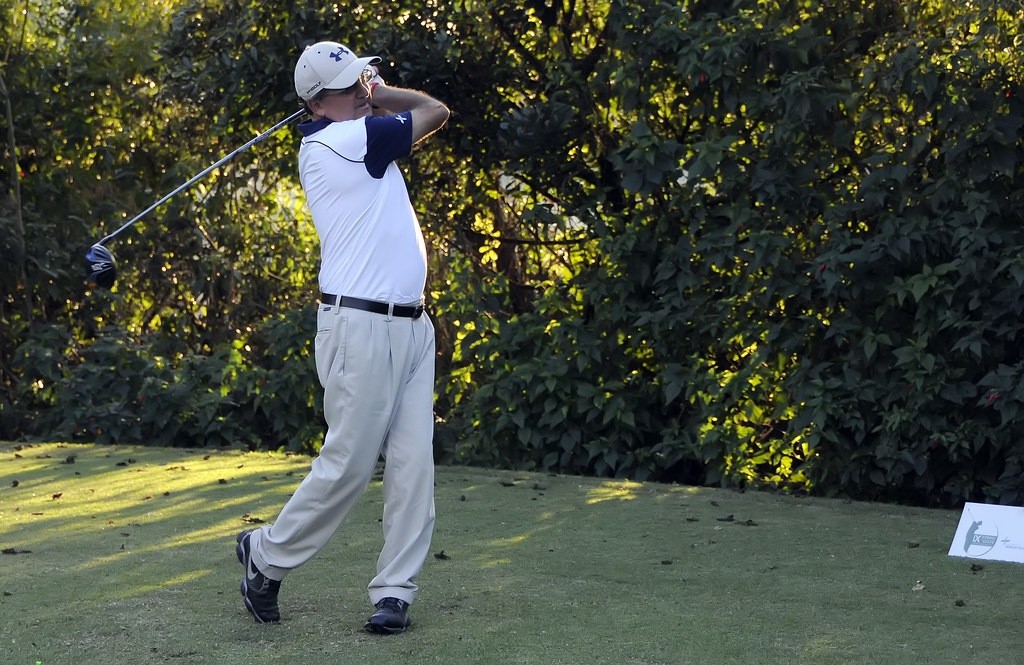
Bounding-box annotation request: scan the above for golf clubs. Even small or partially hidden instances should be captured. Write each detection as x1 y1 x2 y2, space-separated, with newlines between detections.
85 103 313 275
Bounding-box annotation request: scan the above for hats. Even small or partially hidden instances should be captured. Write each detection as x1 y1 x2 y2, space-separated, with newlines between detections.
293 41 382 108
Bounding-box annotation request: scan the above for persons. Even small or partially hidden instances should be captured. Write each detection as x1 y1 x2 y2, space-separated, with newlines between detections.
236 42 451 634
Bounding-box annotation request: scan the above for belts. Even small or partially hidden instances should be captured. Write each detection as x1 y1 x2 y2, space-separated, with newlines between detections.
319 293 426 319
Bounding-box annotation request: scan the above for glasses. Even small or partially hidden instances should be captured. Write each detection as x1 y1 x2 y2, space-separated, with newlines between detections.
319 67 373 98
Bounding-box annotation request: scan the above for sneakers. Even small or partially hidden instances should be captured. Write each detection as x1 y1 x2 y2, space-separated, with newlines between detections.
236 529 282 625
364 597 412 633
